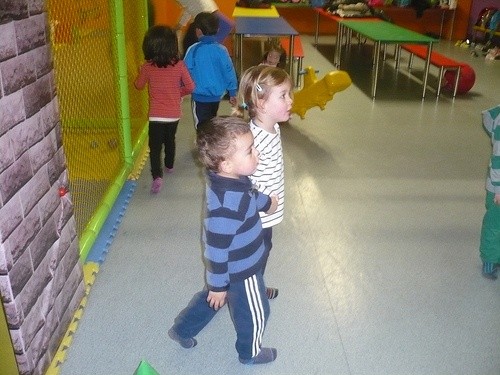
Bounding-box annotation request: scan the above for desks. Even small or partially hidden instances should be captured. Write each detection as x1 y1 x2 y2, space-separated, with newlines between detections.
232 5 280 59
313 7 385 66
336 20 440 97
235 16 300 83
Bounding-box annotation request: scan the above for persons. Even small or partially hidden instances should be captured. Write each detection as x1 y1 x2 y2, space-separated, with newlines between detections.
168 115 278 365
176 0 220 61
132 24 195 195
182 11 241 130
481 105 500 273
230 64 292 300
256 37 287 68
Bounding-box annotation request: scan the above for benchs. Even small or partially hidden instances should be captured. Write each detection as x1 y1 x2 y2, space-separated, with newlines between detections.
472 26 500 48
396 43 464 97
279 35 304 87
